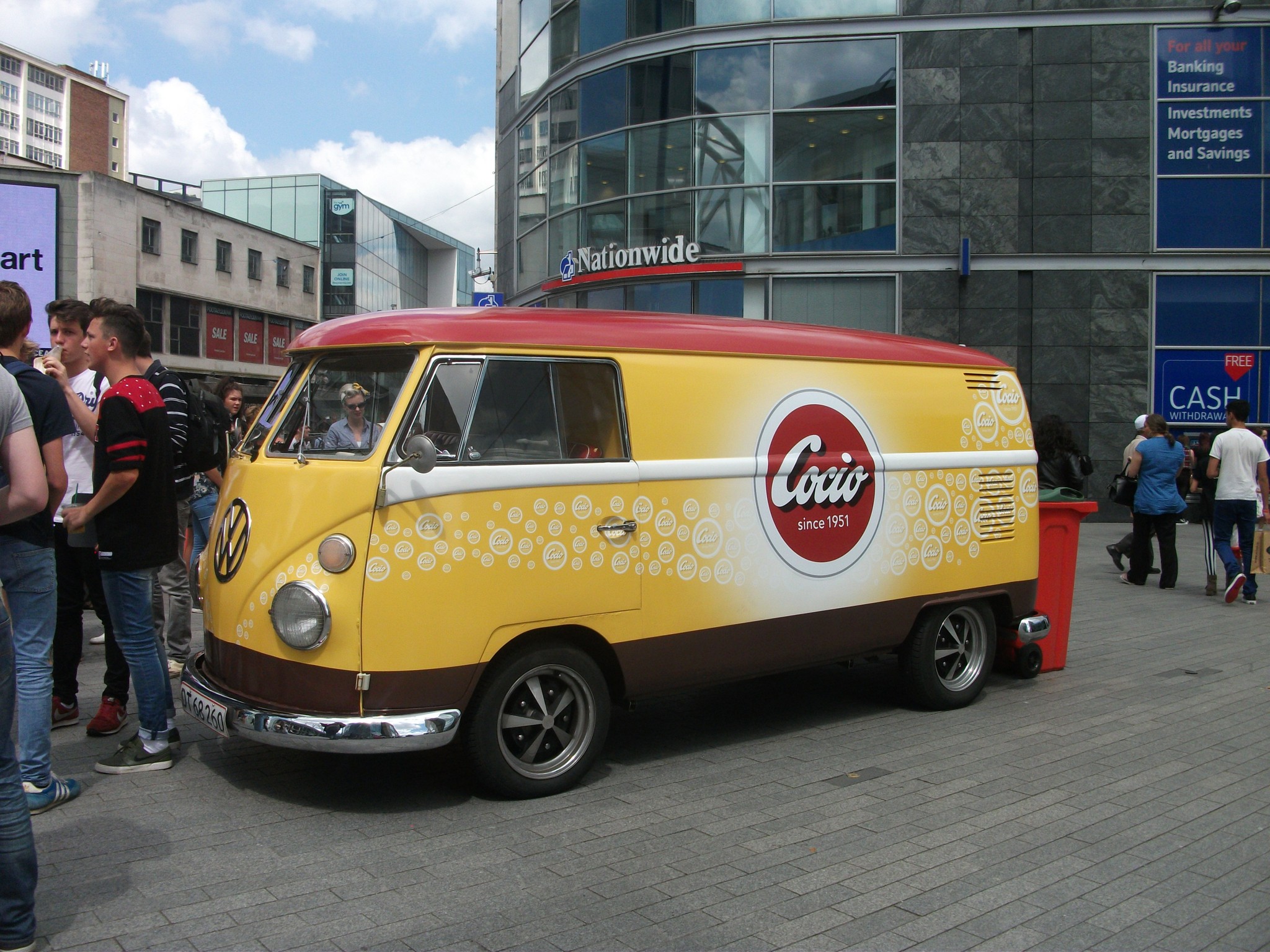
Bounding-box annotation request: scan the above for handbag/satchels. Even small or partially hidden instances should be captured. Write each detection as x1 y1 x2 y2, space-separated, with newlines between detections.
1077 455 1093 475
1250 522 1270 575
1107 461 1139 506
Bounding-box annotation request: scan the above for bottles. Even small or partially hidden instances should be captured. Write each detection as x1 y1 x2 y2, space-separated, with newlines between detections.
48 344 63 362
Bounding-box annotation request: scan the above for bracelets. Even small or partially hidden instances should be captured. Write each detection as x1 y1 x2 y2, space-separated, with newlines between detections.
1262 510 1269 513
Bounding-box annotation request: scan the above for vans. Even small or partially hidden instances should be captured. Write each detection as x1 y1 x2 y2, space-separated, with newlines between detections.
176 301 1052 800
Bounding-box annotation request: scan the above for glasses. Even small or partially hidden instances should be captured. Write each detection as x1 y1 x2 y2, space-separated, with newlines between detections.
345 402 365 410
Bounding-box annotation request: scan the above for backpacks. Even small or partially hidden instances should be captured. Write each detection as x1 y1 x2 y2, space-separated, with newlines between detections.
156 371 232 473
317 438 325 449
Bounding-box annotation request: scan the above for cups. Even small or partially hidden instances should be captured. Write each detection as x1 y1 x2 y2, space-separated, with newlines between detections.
62 502 97 547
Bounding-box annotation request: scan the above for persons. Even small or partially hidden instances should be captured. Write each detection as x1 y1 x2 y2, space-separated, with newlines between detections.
1034 414 1083 490
286 421 310 451
0 280 282 952
1106 400 1270 604
325 383 380 449
314 434 324 449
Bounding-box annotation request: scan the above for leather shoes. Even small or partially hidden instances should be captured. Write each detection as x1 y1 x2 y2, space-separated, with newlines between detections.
1107 544 1124 571
1148 566 1160 574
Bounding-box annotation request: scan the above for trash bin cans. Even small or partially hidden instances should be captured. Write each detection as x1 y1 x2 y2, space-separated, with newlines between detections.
993 500 1097 680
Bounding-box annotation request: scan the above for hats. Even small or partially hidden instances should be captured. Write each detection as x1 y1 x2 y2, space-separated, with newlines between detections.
1134 414 1149 430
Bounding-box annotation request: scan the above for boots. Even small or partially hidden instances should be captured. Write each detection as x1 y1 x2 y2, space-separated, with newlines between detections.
1205 575 1217 595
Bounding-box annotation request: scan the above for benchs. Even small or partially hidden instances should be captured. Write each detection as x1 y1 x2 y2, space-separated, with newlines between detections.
421 431 602 459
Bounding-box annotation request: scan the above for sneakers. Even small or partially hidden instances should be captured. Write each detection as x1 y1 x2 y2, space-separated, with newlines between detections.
94 738 172 772
119 726 182 751
23 770 81 815
85 694 129 736
51 696 79 728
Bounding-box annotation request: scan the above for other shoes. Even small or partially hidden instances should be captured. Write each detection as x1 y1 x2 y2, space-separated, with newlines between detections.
168 659 185 678
192 602 203 613
1175 518 1189 525
1242 595 1256 604
1224 573 1246 603
89 633 105 643
1120 573 1146 586
